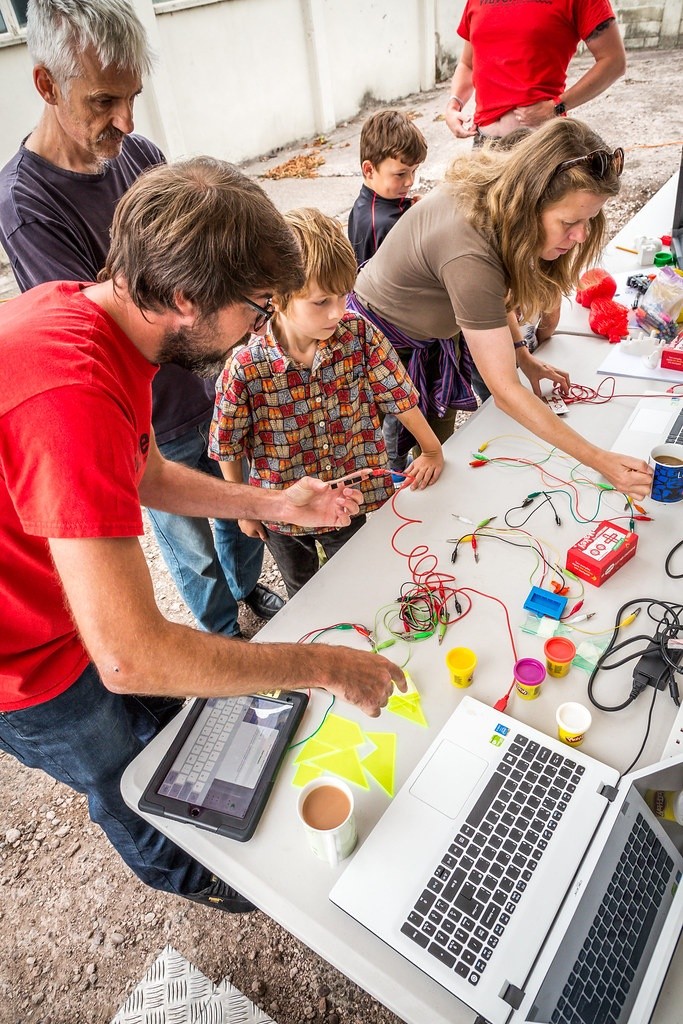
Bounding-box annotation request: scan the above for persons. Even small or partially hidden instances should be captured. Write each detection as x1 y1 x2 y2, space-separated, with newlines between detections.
208 208 444 599
0 157 406 913
443 0 627 153
0 0 286 645
347 119 656 499
348 111 428 273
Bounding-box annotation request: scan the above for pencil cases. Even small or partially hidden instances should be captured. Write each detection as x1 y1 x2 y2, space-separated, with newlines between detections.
634 265 683 336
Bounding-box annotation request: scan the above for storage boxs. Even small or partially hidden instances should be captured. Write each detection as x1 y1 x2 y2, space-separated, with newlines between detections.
661 330 683 371
567 520 638 588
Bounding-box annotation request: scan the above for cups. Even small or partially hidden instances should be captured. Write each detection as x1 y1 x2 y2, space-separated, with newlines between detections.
514 659 546 700
446 646 477 689
649 444 683 504
297 776 358 865
557 704 591 746
545 637 575 677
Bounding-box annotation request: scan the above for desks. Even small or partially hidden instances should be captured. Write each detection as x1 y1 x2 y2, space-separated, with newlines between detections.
115 171 683 1024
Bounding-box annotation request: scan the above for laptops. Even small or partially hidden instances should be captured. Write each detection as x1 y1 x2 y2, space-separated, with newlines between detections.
610 390 683 462
327 694 683 1024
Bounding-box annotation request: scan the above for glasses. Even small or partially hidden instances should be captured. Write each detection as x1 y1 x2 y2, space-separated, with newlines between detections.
236 291 275 332
553 147 625 181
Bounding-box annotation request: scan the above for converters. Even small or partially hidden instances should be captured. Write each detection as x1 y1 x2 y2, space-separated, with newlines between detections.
632 631 683 692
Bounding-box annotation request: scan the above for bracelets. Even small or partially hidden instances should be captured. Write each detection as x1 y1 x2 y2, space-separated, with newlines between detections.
514 339 528 350
449 96 464 112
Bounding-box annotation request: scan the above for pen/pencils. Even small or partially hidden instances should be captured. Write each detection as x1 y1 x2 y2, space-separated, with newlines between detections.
616 247 638 255
636 302 678 343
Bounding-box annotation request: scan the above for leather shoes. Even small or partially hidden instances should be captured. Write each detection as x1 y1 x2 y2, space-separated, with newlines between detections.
240 582 286 620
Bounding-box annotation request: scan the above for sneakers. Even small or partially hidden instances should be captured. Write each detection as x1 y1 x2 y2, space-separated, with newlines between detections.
179 875 256 914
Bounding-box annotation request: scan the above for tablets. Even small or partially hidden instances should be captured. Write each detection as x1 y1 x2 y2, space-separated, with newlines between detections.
138 690 309 843
